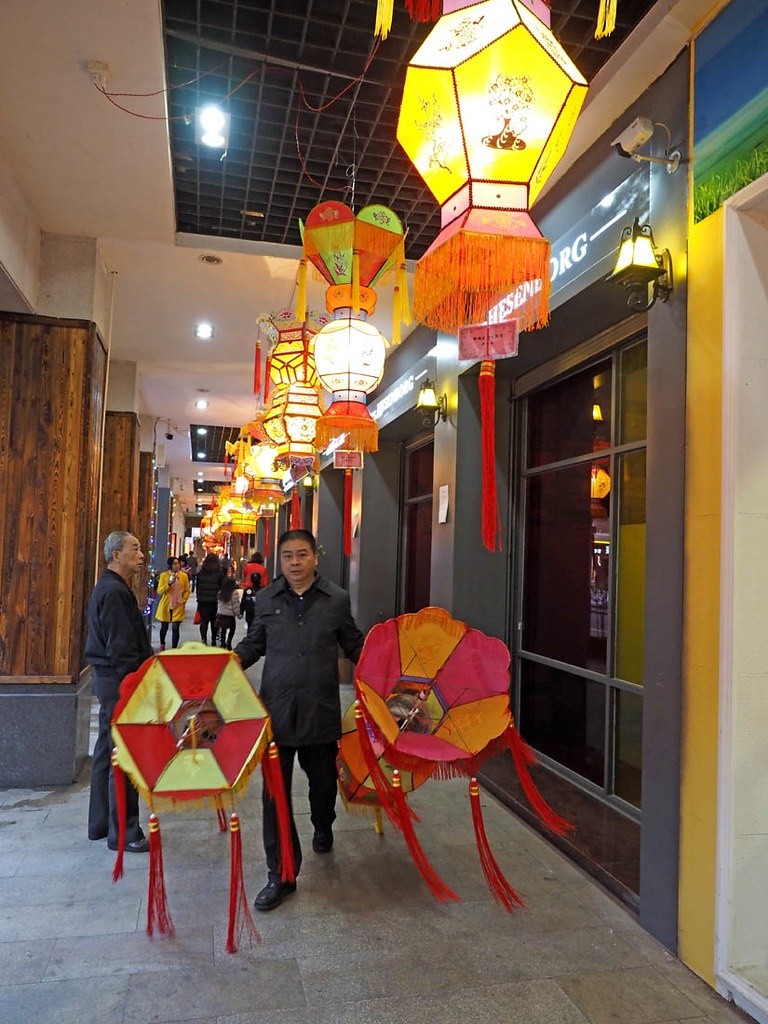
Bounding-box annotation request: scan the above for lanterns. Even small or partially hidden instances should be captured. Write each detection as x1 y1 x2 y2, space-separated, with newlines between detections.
200 200 405 557
111 642 298 954
590 439 611 522
336 607 576 913
374 0 620 551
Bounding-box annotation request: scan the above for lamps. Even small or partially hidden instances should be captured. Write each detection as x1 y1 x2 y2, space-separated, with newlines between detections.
413 378 447 428
605 217 673 313
302 471 317 494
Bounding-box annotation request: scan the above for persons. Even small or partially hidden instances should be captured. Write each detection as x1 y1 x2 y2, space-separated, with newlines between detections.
178 551 248 593
243 552 268 590
195 553 243 651
240 572 266 628
157 557 190 651
83 531 156 853
231 528 366 910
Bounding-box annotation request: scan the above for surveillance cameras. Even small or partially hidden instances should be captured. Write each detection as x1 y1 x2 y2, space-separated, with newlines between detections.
165 432 174 440
609 115 655 159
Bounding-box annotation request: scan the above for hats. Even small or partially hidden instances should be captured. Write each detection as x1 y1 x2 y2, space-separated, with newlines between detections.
251 574 260 581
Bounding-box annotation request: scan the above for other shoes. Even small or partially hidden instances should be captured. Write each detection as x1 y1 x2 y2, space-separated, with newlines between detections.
159 642 165 651
226 642 232 651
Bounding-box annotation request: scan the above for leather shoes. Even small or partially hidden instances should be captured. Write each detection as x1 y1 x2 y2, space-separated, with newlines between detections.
255 877 296 910
108 837 150 852
312 824 333 853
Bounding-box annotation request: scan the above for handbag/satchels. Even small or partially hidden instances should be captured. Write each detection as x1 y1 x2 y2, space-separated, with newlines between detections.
194 610 200 624
